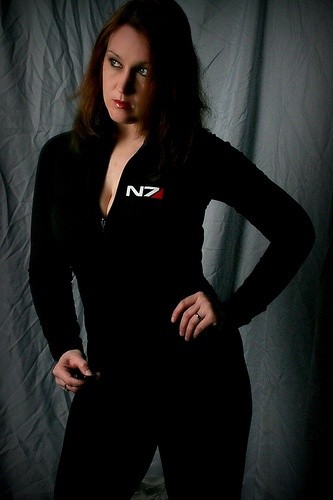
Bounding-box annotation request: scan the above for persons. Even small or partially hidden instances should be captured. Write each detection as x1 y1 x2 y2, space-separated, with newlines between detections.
27 0 319 500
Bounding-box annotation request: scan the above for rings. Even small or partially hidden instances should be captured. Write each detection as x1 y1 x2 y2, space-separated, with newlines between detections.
64 384 70 393
195 312 202 321
